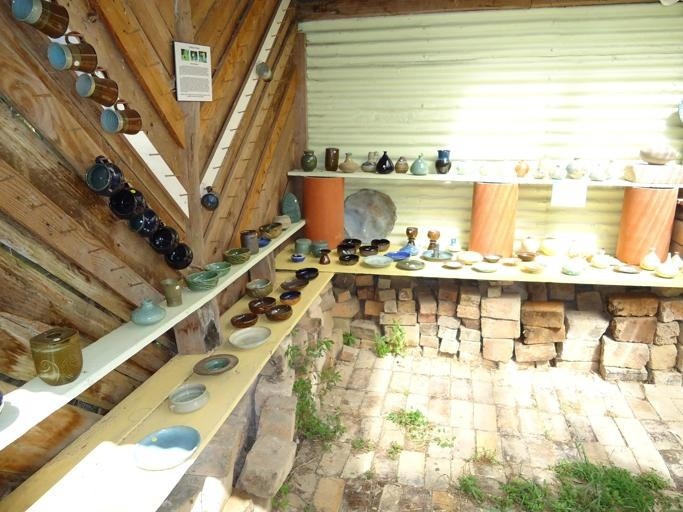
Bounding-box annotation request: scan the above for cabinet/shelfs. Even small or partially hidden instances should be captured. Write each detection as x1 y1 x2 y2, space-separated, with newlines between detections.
273 161 683 306
1 217 335 510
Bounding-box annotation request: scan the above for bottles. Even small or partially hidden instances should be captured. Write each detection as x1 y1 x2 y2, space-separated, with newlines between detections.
410 154 430 175
376 151 394 174
339 153 360 173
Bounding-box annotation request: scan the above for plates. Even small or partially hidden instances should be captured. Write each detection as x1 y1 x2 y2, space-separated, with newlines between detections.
193 353 240 376
473 262 499 274
364 254 393 268
399 260 425 271
521 263 544 274
502 257 522 266
424 250 453 262
445 261 463 269
133 426 202 472
458 251 483 264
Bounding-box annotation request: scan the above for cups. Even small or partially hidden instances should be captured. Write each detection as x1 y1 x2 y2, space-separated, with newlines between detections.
75 67 119 108
100 99 142 136
325 148 339 172
159 277 184 308
46 31 100 74
301 149 318 173
109 183 147 221
150 225 178 256
130 206 162 240
10 0 72 39
86 154 125 194
164 242 195 270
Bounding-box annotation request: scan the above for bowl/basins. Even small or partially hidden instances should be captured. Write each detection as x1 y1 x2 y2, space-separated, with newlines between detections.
278 290 303 306
230 314 259 328
359 246 378 256
205 262 233 276
247 297 277 315
484 254 502 263
281 279 309 291
257 238 271 248
229 326 273 349
184 271 219 292
291 254 305 262
337 245 357 255
266 305 293 321
295 267 319 281
371 239 391 253
259 223 283 239
517 250 537 262
246 279 274 298
166 382 211 414
386 251 411 261
342 239 362 248
222 247 251 264
338 255 359 265
